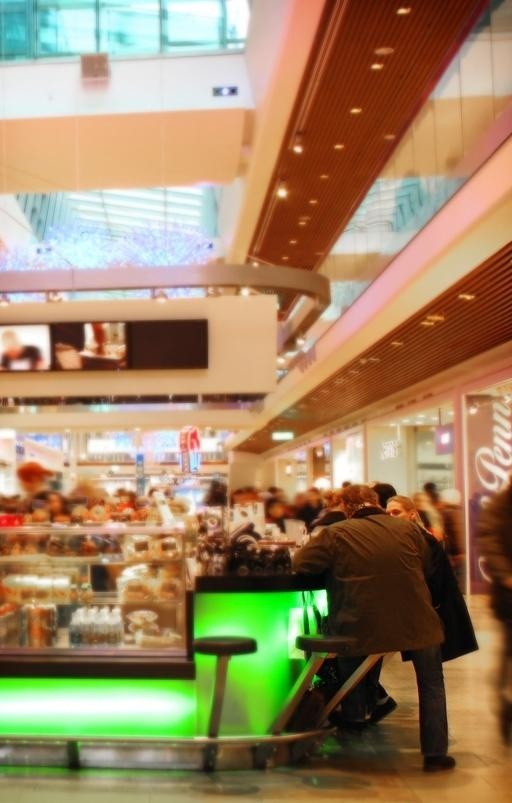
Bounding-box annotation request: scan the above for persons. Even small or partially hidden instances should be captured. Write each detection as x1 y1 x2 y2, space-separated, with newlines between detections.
1 462 190 591
476 471 512 748
204 480 479 776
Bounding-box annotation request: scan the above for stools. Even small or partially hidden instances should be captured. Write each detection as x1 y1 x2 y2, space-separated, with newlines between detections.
190 635 360 774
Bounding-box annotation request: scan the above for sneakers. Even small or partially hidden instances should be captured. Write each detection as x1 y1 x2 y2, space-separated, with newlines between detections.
367 697 396 722
425 753 455 771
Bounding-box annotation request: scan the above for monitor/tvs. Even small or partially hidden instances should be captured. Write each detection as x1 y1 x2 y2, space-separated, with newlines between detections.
0 319 208 373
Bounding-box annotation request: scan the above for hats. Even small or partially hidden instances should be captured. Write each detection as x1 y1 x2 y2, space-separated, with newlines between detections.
18 462 43 480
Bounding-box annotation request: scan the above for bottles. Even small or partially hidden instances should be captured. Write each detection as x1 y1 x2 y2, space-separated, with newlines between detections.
69 607 125 648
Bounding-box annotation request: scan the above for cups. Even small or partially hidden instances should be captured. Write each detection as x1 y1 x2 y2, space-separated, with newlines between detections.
285 520 307 543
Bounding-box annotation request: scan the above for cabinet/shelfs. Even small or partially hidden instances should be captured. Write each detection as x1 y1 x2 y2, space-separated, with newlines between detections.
0 524 191 659
